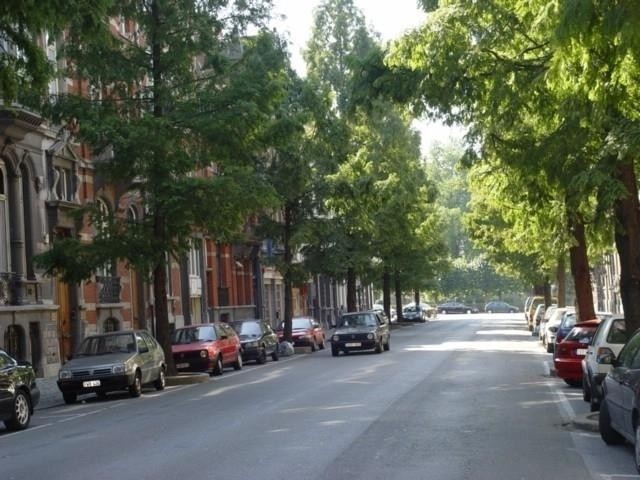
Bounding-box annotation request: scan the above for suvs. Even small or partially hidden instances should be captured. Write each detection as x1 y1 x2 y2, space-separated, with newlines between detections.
330 312 390 356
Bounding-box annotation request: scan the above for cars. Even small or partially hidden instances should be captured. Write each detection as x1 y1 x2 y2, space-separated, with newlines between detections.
485 302 519 313
373 302 434 322
228 319 279 364
523 297 640 471
436 302 479 314
0 347 39 431
274 317 325 352
171 322 244 374
56 328 165 402
